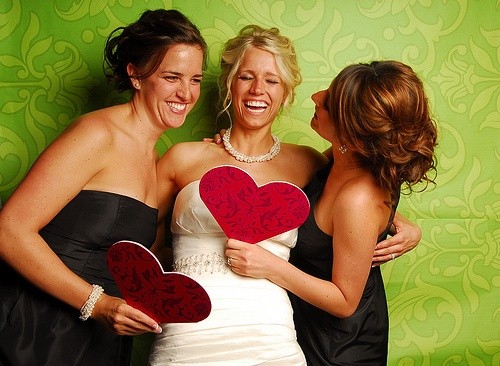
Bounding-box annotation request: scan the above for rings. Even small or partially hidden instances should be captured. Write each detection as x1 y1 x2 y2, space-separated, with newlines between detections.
391 254 395 260
227 258 231 266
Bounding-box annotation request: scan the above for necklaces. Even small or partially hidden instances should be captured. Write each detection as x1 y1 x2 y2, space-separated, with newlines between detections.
222 127 280 163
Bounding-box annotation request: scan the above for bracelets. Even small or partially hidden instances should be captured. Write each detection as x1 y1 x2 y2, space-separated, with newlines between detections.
77 283 105 322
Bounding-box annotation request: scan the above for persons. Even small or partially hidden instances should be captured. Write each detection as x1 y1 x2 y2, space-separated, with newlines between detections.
152 24 422 366
0 9 207 366
224 60 438 366
